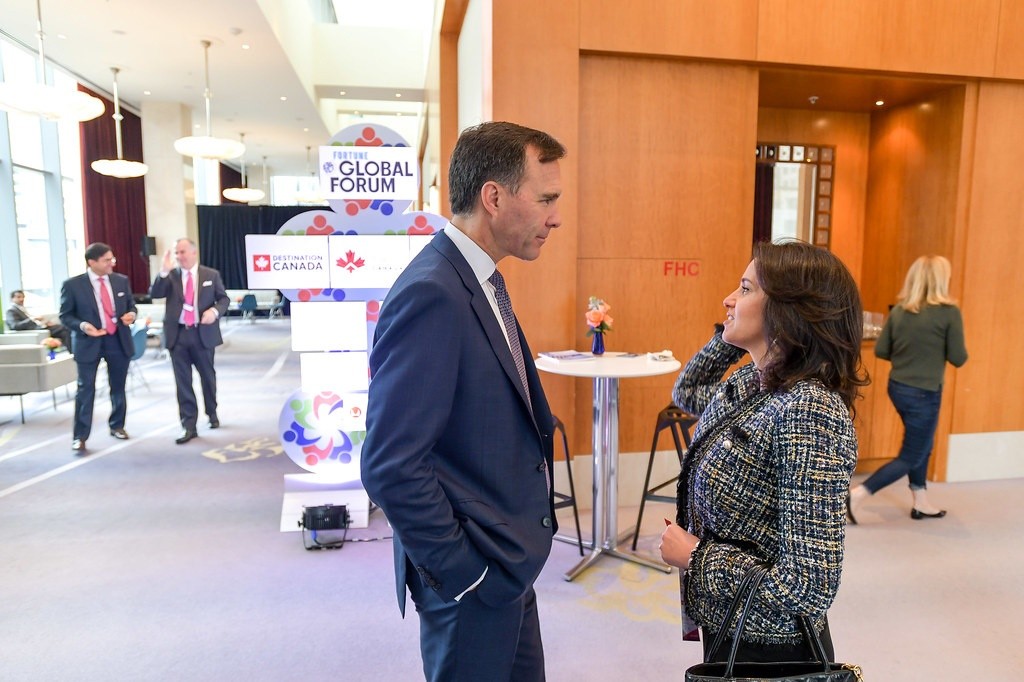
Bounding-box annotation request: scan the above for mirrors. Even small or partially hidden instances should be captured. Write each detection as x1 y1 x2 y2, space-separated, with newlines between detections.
752 141 836 256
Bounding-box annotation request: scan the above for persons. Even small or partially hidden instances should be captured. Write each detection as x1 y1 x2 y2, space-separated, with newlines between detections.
150 238 231 444
6 291 72 354
360 121 567 682
59 243 138 451
847 255 968 526
657 241 872 663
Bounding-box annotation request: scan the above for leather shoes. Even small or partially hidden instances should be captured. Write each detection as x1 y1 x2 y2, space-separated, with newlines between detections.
176 432 197 444
210 421 219 428
72 439 86 450
110 429 129 439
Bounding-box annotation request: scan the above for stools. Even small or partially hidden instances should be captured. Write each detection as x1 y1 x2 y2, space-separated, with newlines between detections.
550 414 584 558
631 401 700 550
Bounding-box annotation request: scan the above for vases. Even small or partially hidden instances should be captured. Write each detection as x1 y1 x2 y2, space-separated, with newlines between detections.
591 323 605 356
51 349 55 360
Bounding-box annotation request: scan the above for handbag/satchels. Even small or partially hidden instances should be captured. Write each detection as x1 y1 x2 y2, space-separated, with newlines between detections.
683 565 862 682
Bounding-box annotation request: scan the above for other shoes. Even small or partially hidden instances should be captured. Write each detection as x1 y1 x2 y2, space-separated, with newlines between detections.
911 508 947 520
847 489 857 524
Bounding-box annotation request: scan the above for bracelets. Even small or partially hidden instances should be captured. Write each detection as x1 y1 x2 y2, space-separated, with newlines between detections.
688 541 700 565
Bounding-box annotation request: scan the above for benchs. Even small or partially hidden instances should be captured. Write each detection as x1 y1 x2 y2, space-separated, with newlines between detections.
134 304 166 359
224 290 277 319
0 313 77 425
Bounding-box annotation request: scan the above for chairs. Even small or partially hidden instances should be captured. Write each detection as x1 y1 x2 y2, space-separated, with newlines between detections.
99 328 148 400
269 295 285 319
238 295 257 319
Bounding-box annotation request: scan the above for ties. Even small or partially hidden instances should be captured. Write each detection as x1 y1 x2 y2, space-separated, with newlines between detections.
183 271 194 327
488 268 551 501
98 278 116 335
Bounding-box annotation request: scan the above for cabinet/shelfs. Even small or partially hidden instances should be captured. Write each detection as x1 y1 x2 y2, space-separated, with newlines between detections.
849 339 905 474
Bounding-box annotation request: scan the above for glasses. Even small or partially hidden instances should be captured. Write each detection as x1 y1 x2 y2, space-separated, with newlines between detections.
94 256 116 265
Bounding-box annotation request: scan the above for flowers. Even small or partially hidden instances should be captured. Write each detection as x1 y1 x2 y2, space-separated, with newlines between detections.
40 337 63 349
585 295 614 337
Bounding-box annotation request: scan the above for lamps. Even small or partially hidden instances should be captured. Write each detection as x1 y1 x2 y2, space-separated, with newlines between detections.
0 0 105 123
90 67 148 178
174 40 247 160
222 133 265 203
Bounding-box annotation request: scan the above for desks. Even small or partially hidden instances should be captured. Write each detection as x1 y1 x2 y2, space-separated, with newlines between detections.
538 353 681 581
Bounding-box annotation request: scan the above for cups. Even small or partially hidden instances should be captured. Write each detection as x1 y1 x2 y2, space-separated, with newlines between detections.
863 311 884 340
